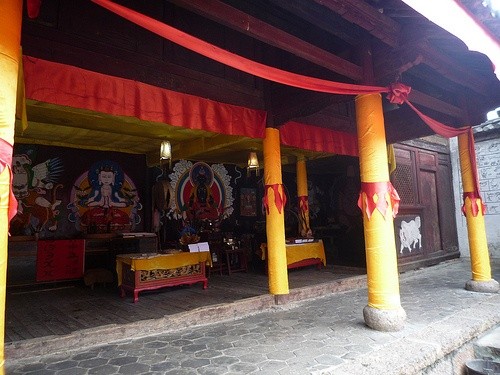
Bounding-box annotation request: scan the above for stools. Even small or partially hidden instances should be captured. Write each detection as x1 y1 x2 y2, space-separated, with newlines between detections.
223 250 249 276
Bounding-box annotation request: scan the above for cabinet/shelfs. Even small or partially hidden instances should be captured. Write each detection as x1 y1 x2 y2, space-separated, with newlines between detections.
7 233 159 297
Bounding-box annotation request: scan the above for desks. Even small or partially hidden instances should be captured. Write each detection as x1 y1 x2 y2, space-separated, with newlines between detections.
116 248 209 302
259 238 326 272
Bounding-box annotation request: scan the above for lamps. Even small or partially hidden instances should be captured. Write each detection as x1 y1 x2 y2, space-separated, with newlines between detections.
159 136 172 170
246 151 260 178
